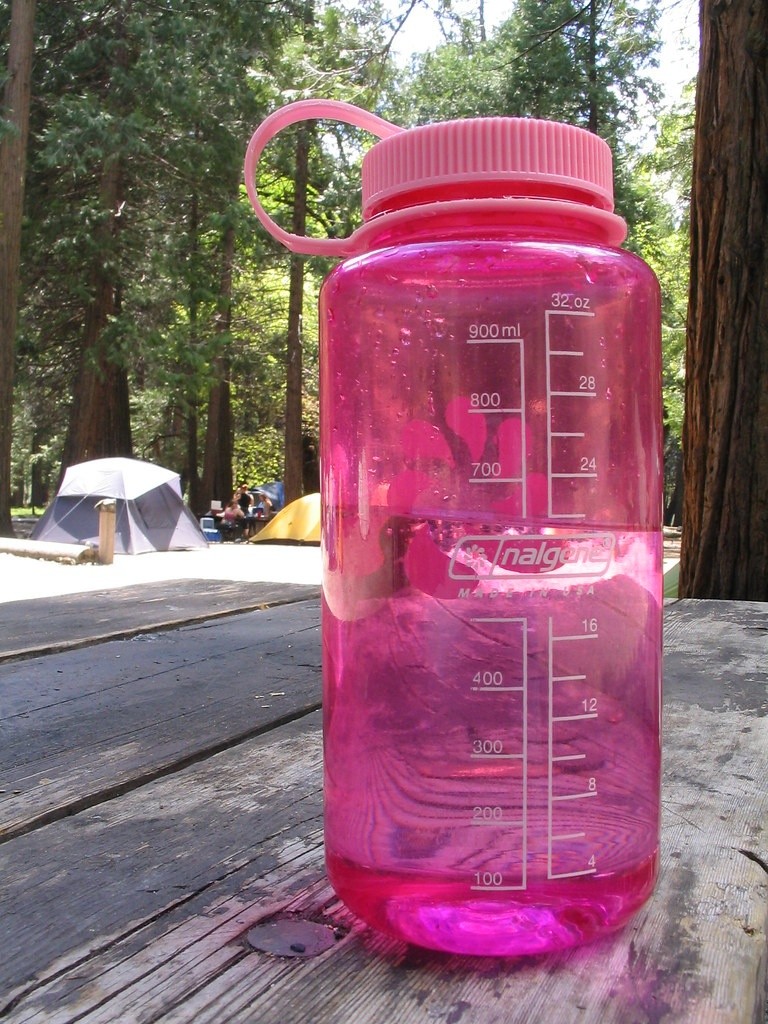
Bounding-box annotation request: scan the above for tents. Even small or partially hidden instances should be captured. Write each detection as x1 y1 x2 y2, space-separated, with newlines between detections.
249 493 321 545
29 456 211 555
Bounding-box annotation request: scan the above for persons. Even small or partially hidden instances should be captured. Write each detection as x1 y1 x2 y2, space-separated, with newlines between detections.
221 501 245 542
258 493 275 526
233 484 254 538
246 494 257 535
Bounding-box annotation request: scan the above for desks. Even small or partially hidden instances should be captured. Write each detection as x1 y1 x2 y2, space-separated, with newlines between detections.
1 580 768 1024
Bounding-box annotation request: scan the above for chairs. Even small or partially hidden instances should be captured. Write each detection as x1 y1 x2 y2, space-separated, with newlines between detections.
205 514 274 543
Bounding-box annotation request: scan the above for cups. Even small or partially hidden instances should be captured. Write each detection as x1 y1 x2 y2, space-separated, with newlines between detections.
242 99 659 954
